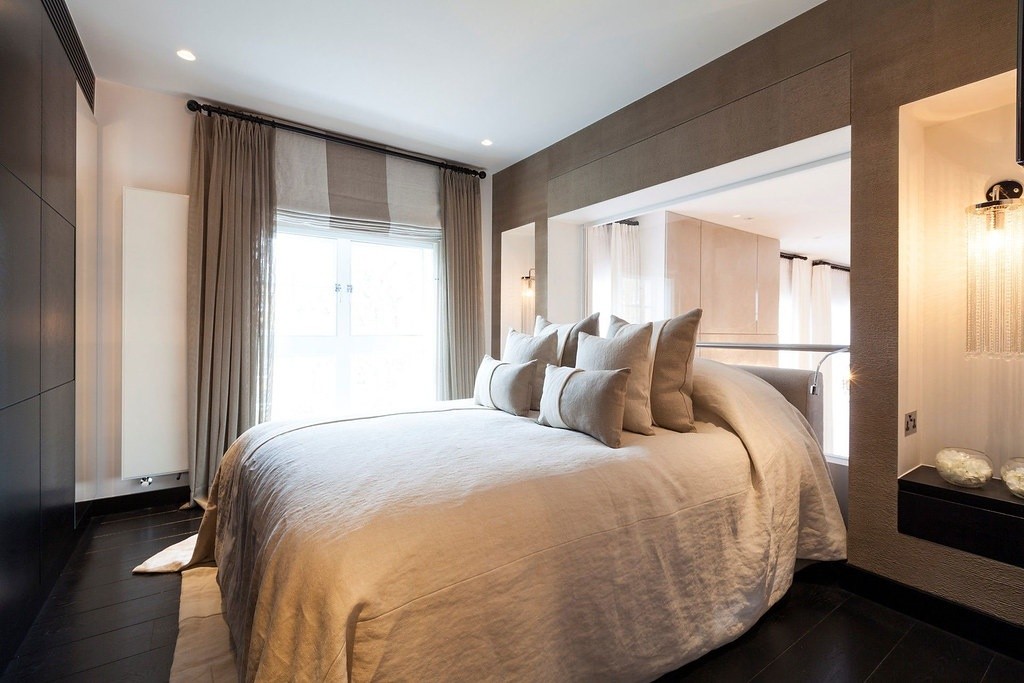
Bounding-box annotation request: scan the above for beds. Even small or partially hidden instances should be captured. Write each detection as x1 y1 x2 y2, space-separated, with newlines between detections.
127 362 849 683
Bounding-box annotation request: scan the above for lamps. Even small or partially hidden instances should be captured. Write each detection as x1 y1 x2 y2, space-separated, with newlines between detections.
521 268 535 298
964 180 1023 362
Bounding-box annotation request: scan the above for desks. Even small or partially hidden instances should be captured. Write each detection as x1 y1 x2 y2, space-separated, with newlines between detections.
897 465 1024 568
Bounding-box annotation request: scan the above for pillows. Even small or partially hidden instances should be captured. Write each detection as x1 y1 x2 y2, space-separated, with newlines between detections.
504 327 559 411
536 363 632 449
608 307 699 434
534 311 600 366
575 321 656 436
473 353 539 417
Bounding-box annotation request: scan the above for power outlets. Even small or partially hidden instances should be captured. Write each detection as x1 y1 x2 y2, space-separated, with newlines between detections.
905 410 918 438
